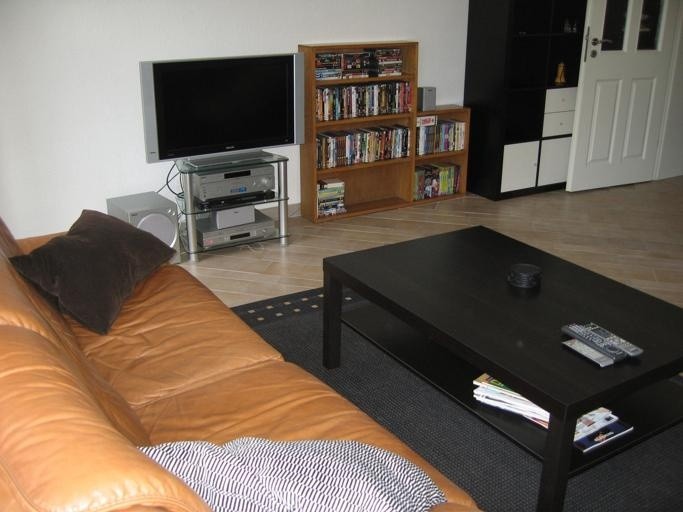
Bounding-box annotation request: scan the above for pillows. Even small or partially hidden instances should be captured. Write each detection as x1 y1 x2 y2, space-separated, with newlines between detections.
138 438 449 512
9 208 179 335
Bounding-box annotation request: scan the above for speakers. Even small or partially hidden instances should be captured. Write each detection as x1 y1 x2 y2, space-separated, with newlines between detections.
418 87 436 112
106 190 181 265
209 205 256 230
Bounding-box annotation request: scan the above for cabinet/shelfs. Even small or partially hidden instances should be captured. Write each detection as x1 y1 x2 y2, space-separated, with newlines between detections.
500 3 591 196
414 114 469 204
173 149 292 265
315 43 415 219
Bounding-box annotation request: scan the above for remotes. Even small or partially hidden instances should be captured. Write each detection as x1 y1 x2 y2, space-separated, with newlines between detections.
561 339 614 368
560 322 628 362
583 322 644 357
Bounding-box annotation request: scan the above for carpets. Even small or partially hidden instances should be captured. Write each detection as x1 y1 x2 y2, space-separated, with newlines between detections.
229 284 681 512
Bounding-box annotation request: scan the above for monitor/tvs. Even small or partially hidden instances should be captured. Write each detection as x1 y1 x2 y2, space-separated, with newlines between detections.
139 52 305 167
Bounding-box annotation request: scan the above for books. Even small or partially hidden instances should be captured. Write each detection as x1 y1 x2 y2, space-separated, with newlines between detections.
316 47 412 218
415 116 465 199
473 371 634 453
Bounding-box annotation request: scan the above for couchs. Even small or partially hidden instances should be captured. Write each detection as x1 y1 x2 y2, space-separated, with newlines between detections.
1 217 484 512
